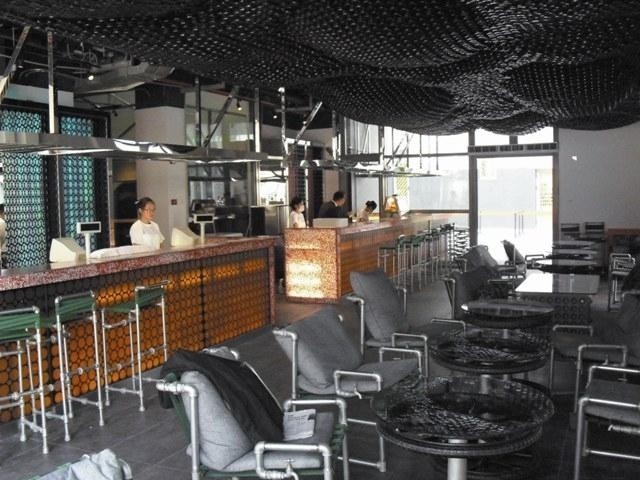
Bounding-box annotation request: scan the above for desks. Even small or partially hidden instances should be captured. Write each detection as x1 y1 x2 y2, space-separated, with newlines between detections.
546 287 630 411
373 367 558 480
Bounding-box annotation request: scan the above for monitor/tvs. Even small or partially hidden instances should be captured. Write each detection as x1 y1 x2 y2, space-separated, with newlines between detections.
49 237 84 262
171 226 201 247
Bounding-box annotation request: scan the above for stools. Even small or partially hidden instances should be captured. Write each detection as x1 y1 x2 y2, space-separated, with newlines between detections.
18 288 110 442
0 303 52 456
97 278 172 416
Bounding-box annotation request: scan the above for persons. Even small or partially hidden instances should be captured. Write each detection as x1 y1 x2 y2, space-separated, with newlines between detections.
129 198 165 252
0 204 8 250
278 196 305 295
319 191 345 218
357 201 377 222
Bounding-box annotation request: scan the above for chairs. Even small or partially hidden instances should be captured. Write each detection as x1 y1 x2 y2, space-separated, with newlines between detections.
152 345 353 479
433 322 553 377
341 267 470 382
273 303 425 475
375 228 638 337
572 362 636 479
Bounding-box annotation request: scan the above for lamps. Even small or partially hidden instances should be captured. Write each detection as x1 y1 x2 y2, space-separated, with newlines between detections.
236 97 243 111
270 109 277 119
